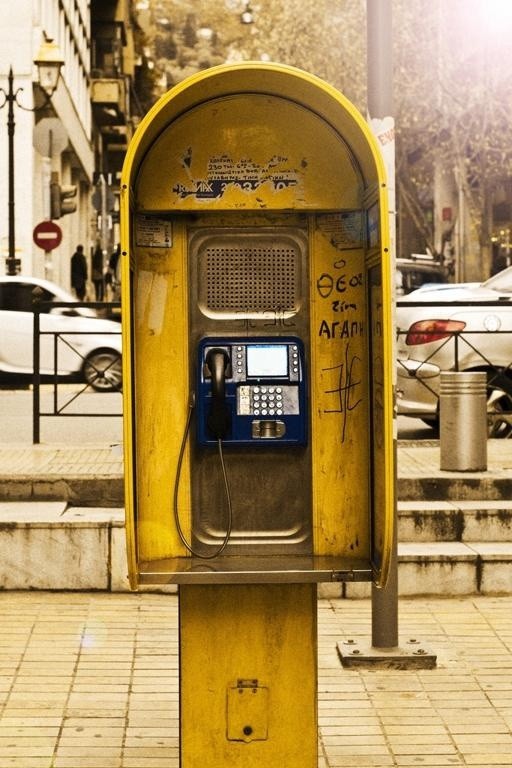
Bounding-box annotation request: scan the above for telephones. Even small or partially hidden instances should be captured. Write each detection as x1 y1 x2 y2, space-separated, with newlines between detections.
205 348 232 438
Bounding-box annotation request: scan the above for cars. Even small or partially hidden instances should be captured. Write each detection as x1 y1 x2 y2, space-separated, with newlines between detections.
396 264 512 439
0 276 126 392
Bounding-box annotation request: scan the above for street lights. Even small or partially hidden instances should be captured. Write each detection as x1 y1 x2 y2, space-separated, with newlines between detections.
1 36 65 277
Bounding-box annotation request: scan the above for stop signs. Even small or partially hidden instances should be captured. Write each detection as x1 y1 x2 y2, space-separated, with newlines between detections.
33 220 62 254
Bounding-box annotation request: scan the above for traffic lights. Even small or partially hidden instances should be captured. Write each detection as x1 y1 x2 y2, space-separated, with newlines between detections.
51 185 77 219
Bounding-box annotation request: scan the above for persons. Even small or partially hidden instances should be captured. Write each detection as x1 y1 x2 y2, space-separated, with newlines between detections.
109 241 121 277
69 244 89 305
88 238 108 303
